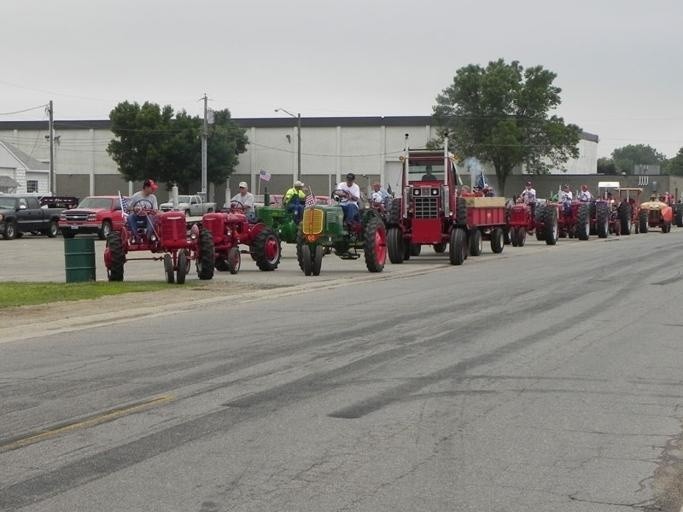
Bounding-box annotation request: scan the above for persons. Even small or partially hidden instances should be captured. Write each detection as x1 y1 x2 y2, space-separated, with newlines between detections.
461 181 592 215
336 173 360 226
282 181 306 224
127 179 158 245
231 182 256 222
368 183 388 204
422 164 436 181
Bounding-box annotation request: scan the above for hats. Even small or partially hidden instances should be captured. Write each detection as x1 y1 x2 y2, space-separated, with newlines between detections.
525 182 531 185
144 179 158 191
347 173 355 180
372 184 381 188
295 181 304 187
582 185 587 189
562 183 568 188
239 182 247 187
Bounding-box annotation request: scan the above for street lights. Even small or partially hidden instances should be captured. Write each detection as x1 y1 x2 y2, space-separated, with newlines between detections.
274 107 302 181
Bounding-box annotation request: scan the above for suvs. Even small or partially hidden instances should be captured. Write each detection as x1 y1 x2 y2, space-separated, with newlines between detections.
0 194 69 239
58 195 133 238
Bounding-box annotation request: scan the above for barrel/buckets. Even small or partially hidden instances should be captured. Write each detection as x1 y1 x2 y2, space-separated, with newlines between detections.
63 235 96 283
